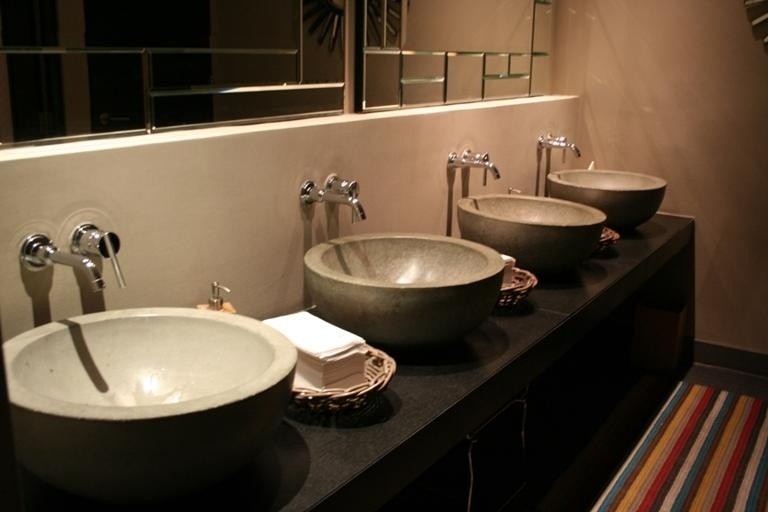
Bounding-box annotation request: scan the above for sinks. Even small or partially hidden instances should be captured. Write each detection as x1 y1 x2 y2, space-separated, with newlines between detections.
546 167 669 230
455 194 608 270
2 307 299 499
304 232 507 345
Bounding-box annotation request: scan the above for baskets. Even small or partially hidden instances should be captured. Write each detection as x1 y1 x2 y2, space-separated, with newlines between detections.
588 227 621 258
493 267 538 316
292 343 397 417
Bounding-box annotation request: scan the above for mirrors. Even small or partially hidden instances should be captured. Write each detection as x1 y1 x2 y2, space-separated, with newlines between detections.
1 0 355 147
360 0 556 112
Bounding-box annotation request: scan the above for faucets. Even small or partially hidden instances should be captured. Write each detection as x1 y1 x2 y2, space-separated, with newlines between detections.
18 234 107 295
299 180 367 224
537 135 582 160
446 153 502 181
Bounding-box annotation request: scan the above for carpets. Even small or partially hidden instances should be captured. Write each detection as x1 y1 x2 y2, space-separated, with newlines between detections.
580 377 768 511
